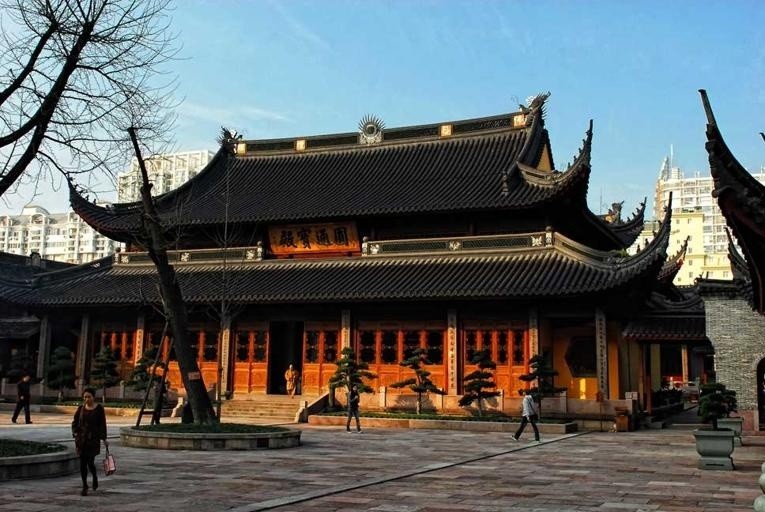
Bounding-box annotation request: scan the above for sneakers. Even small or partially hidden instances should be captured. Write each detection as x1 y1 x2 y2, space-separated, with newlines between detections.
510 435 516 441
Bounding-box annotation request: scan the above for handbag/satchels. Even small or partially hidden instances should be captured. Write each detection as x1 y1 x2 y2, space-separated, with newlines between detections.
102 446 115 475
533 414 538 420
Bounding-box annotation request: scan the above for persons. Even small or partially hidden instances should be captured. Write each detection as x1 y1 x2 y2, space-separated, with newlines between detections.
284 360 299 395
346 384 363 434
511 388 540 443
11 373 34 424
182 401 194 424
71 387 109 496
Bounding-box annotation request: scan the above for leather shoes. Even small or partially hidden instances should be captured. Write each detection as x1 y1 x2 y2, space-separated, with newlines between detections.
92 479 98 490
81 486 88 496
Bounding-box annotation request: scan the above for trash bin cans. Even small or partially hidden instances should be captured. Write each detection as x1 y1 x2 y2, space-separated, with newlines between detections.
614 406 628 432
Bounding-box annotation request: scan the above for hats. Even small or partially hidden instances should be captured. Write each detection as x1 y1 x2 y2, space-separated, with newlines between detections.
520 389 530 393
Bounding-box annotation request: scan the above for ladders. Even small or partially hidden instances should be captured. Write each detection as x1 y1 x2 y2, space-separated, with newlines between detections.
136 322 174 427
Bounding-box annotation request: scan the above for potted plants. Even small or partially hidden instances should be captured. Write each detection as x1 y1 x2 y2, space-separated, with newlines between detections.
693 382 744 470
